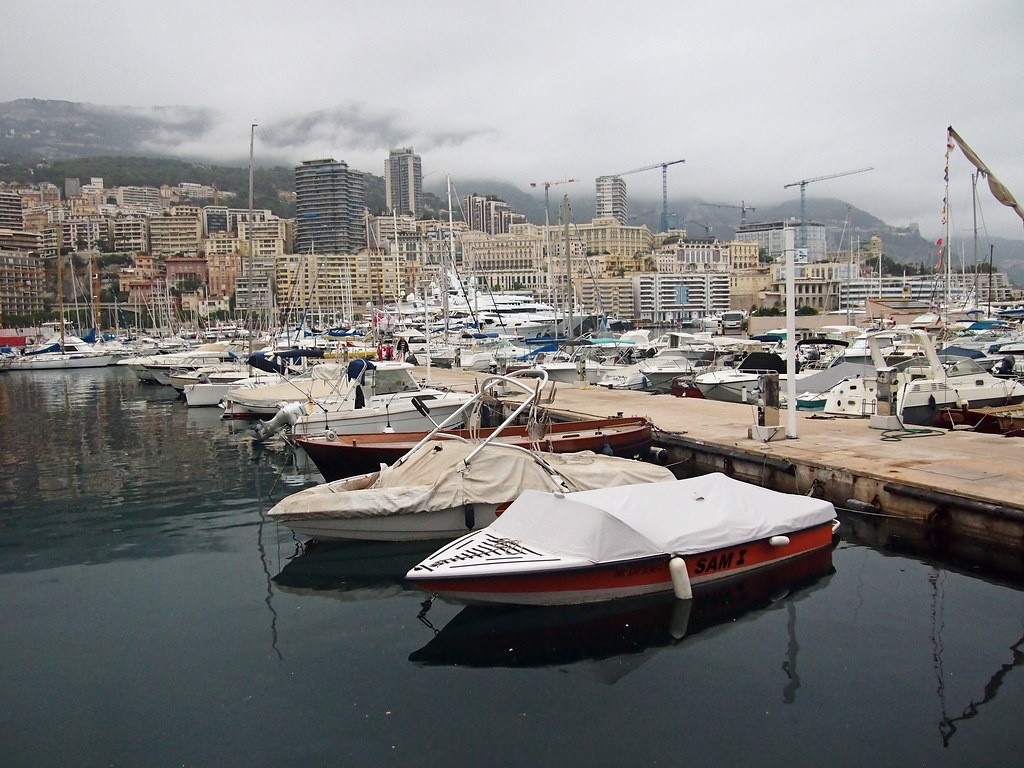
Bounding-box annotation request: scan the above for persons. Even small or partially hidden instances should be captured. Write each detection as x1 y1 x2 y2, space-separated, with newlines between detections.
397 337 408 361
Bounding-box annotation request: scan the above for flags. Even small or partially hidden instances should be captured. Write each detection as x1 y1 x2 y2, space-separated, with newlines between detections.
941 141 955 224
935 239 942 268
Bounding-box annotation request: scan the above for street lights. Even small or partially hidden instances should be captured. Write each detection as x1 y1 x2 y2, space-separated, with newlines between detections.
248 123 260 378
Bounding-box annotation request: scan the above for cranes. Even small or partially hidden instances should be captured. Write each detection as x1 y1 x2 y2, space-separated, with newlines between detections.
530 178 578 224
783 164 875 247
698 201 757 227
614 159 685 233
684 218 713 238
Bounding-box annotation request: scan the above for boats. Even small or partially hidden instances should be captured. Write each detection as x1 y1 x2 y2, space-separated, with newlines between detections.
0 172 534 403
295 416 666 483
523 297 1024 436
261 364 680 540
254 359 489 445
405 468 842 608
